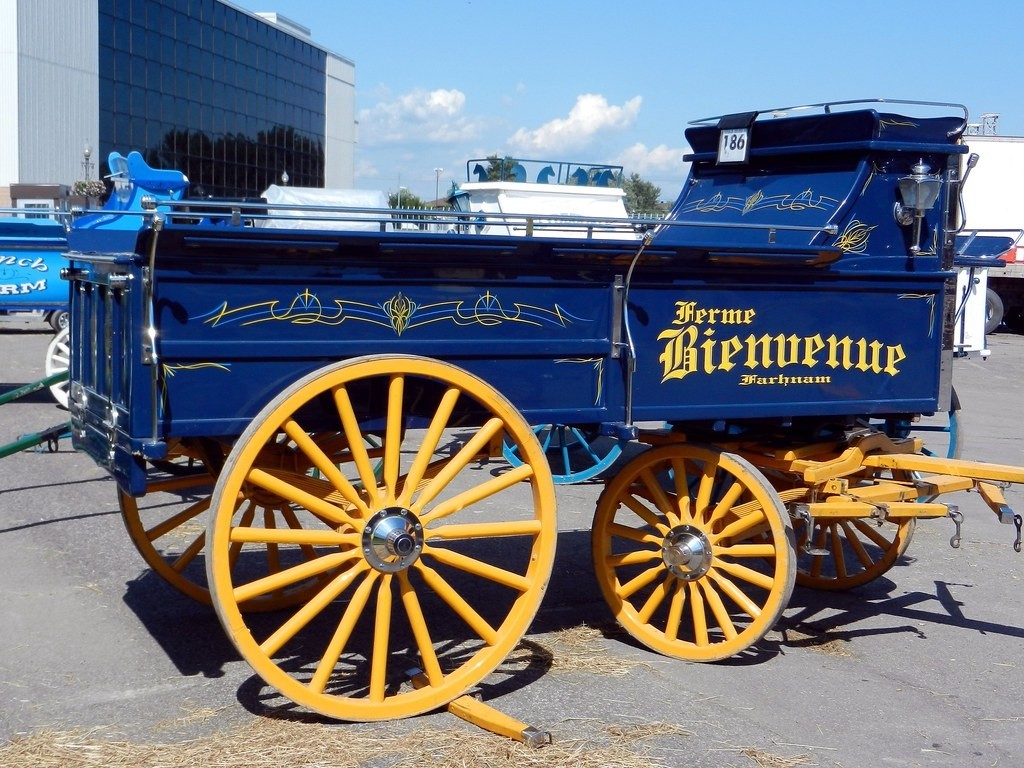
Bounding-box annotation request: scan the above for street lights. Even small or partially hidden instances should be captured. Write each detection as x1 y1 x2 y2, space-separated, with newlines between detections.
81 142 94 208
435 167 444 222
